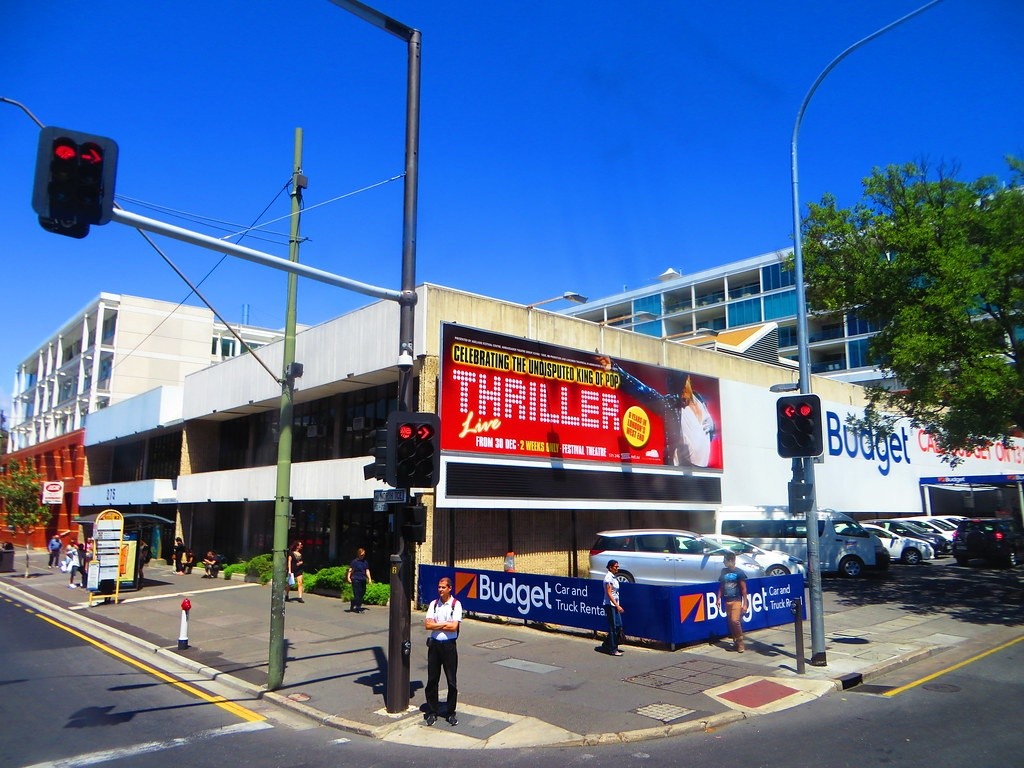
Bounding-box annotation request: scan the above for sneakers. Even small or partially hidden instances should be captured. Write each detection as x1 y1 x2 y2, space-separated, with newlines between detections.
447 716 459 725
426 714 436 726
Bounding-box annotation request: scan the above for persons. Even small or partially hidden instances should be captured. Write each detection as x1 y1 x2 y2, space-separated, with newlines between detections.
48 535 62 568
177 550 195 576
347 548 371 615
425 578 463 726
597 354 715 469
65 537 149 604
284 541 305 603
716 552 747 653
203 551 220 578
604 561 624 656
173 537 184 574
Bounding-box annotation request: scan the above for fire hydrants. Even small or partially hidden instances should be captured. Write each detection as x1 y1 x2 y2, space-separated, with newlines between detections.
179 599 192 649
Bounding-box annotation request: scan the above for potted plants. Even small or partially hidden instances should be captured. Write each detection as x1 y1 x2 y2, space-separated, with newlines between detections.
310 564 349 598
342 582 390 606
244 554 273 585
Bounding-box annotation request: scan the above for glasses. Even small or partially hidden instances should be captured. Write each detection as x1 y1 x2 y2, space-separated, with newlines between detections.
361 555 366 556
615 566 619 569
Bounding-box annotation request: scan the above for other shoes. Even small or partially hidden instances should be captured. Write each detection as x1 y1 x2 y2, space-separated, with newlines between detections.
55 565 59 568
48 565 51 568
70 583 76 589
298 598 304 603
602 643 622 656
201 575 214 578
285 595 290 602
726 646 744 652
172 571 184 575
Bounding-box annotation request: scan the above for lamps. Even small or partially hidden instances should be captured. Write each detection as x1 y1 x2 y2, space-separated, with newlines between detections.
524 290 719 340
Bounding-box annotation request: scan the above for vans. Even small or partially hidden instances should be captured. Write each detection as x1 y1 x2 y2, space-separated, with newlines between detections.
715 507 891 578
586 529 766 583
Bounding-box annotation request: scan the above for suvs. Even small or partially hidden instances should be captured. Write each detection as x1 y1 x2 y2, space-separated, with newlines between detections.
951 515 1023 567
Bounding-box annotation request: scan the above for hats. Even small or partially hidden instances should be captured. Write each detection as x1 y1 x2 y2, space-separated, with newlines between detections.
666 370 688 395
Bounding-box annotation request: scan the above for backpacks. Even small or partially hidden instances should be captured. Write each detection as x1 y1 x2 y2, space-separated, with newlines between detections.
217 554 227 562
434 598 459 638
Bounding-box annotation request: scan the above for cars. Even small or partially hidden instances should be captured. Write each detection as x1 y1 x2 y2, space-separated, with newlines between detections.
834 523 936 566
676 537 807 578
860 518 951 558
897 515 971 543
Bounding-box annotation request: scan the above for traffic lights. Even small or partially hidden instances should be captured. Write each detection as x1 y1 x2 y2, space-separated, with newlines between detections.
787 480 813 515
776 394 823 458
386 414 442 490
399 505 428 542
33 125 121 227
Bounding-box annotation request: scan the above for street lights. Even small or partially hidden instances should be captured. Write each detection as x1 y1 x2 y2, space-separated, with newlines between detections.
769 382 826 666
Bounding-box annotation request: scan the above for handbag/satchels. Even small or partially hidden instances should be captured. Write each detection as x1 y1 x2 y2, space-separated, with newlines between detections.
142 545 152 563
